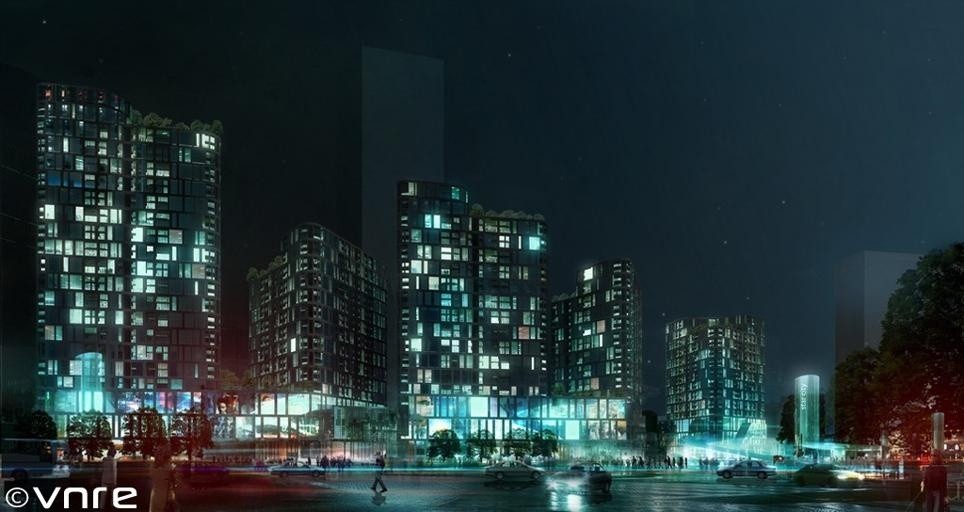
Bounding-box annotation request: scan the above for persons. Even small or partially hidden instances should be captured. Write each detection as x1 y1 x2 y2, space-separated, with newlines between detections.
370 449 388 493
698 456 721 470
143 444 183 512
276 456 297 466
306 455 353 472
99 449 118 509
625 455 687 469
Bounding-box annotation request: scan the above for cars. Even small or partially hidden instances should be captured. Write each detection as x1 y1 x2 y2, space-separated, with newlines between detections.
484 459 544 480
716 460 775 479
269 460 324 478
0 452 70 484
545 461 612 496
793 463 864 488
